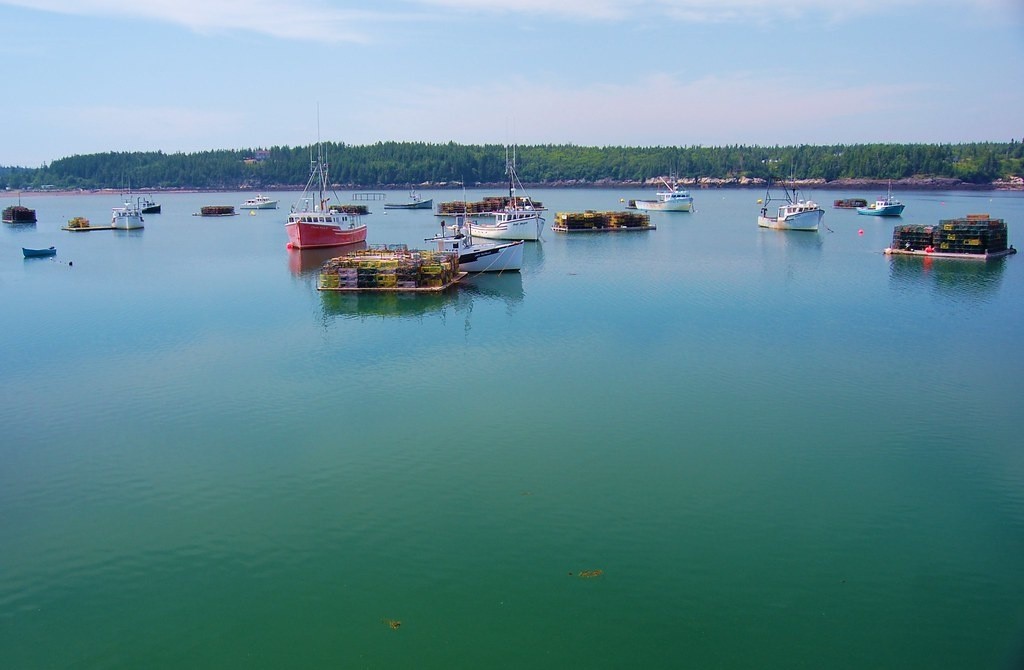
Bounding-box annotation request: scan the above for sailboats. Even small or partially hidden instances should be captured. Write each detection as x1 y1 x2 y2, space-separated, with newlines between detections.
628 175 694 212
855 178 906 217
284 239 368 279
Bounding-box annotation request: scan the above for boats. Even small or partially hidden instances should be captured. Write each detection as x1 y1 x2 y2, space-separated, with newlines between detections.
463 145 546 241
424 187 524 274
832 198 869 211
110 175 146 230
140 193 161 214
757 154 826 232
22 246 57 257
383 197 434 210
283 102 368 251
239 193 278 209
